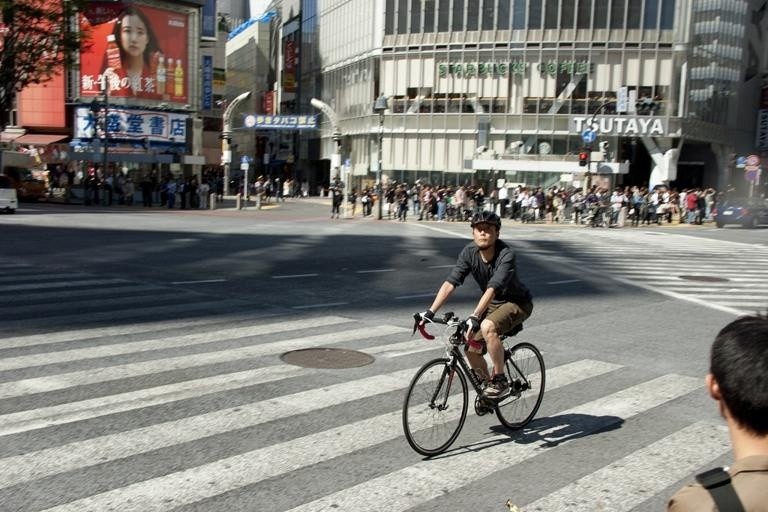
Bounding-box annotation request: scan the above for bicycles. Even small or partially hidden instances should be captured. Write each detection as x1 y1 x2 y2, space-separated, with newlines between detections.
400 308 559 458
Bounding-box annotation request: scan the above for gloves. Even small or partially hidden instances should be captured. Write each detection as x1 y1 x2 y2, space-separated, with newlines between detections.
414 310 434 326
463 315 481 341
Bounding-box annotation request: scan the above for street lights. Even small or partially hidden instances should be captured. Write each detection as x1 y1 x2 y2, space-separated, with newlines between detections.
92 75 113 185
374 91 390 223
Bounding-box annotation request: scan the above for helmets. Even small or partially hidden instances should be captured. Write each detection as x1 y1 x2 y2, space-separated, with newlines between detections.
470 210 502 230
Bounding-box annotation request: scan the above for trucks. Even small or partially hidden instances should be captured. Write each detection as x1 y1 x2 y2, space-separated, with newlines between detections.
0 148 46 203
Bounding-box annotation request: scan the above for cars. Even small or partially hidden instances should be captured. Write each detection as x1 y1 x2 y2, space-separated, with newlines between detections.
1 173 19 216
713 196 768 229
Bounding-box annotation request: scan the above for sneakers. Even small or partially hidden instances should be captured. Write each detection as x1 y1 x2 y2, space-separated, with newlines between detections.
483 379 511 400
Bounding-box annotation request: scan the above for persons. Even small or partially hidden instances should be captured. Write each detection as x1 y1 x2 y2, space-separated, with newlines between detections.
54 165 309 211
418 209 535 402
98 6 167 100
665 308 768 512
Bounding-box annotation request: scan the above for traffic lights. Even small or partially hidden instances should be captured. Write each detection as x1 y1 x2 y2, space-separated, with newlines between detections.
579 152 587 166
599 141 610 157
728 153 737 168
635 99 667 112
